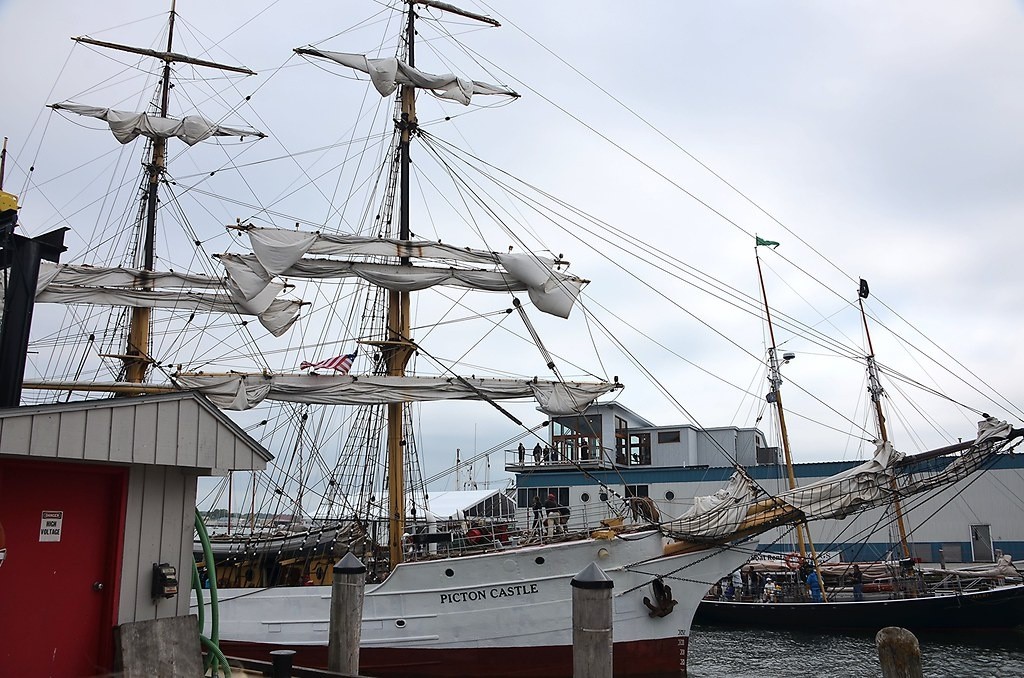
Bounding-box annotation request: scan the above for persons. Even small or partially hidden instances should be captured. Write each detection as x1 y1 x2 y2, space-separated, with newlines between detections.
543 446 549 464
531 494 570 536
518 443 526 462
720 557 825 603
850 564 864 600
581 439 588 459
534 443 542 465
551 446 558 461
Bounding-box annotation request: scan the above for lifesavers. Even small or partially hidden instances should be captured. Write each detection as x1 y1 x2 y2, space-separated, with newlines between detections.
786 552 803 569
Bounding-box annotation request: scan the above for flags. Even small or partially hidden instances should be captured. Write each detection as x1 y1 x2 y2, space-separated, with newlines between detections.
299 351 356 374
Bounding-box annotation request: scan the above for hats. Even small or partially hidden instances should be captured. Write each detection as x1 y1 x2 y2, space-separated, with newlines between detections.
766 578 771 581
548 494 555 497
